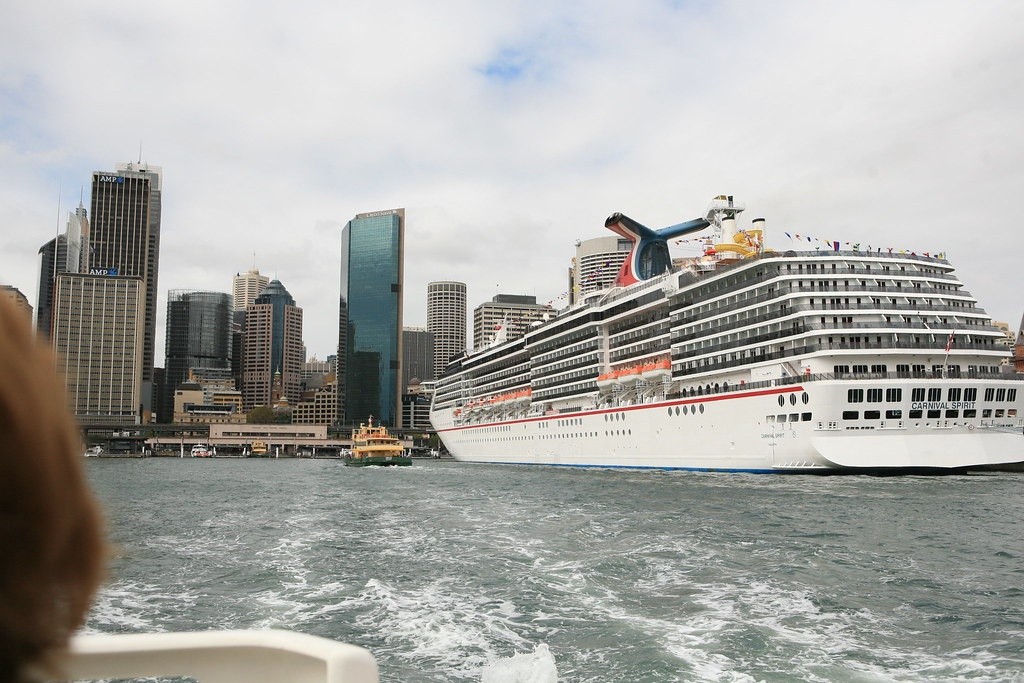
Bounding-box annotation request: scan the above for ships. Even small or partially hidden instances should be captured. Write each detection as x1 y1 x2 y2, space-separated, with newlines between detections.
428 195 1024 475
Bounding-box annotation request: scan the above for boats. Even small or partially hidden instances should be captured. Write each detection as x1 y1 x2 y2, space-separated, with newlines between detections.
192 445 216 458
84 445 102 457
340 414 412 467
250 439 270 458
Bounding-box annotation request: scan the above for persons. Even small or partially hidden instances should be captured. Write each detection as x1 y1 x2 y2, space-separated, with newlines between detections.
345 453 364 462
0 285 111 683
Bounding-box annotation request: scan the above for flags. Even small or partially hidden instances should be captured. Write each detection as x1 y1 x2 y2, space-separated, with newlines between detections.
945 331 954 352
